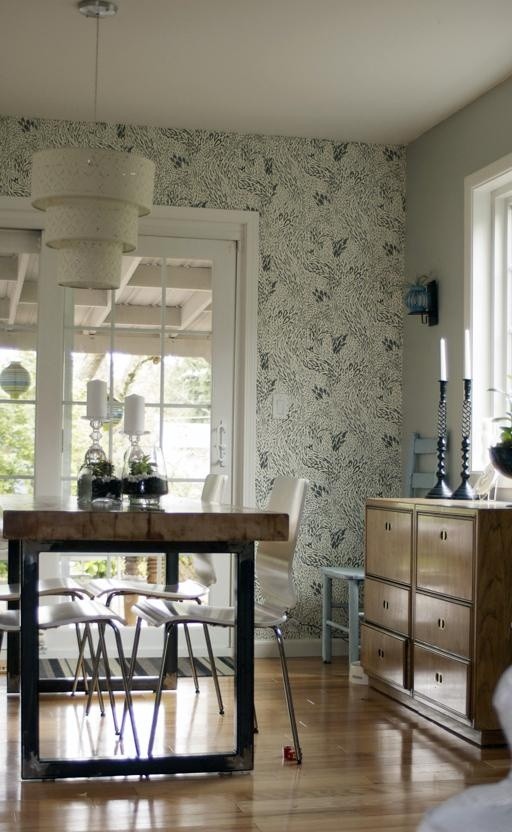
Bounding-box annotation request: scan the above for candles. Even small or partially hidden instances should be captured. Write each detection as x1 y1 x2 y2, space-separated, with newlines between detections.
125 393 145 435
87 380 108 422
439 337 447 384
464 329 470 381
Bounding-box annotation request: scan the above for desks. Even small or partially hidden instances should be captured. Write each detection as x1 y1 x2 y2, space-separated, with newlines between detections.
2 494 288 782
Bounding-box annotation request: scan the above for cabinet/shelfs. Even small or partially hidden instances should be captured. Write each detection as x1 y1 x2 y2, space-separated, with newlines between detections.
362 495 511 748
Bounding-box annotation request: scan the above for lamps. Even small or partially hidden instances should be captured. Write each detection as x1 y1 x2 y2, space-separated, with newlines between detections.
29 1 156 292
402 276 441 326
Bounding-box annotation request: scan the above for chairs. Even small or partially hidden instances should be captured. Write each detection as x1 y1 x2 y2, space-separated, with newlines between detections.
78 472 229 716
318 432 449 673
119 475 309 777
0 601 141 780
0 576 107 720
